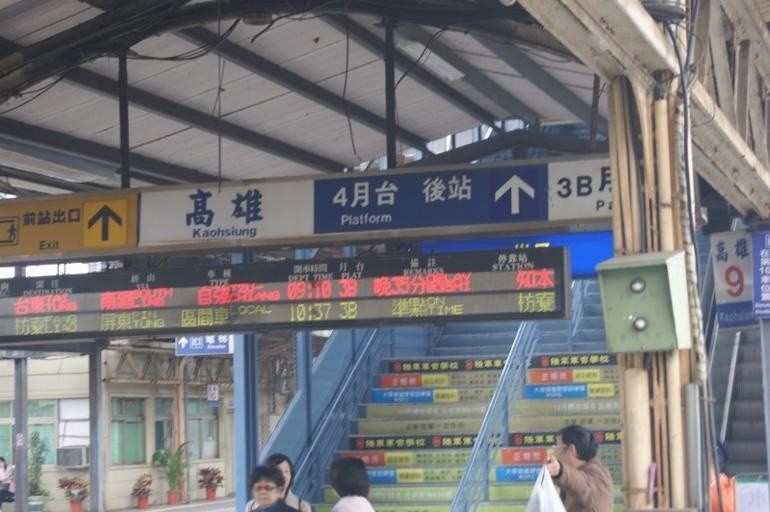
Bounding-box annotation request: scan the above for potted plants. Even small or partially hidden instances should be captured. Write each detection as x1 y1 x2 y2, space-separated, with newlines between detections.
197 468 223 501
61 478 88 512
26 432 51 512
131 474 153 510
156 441 189 505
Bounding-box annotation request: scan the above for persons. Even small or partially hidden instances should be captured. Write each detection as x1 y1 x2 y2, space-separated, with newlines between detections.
0 456 16 510
249 464 302 512
544 425 613 512
328 457 376 512
246 453 312 511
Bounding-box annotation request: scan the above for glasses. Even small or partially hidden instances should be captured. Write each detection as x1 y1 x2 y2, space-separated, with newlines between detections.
253 485 275 493
